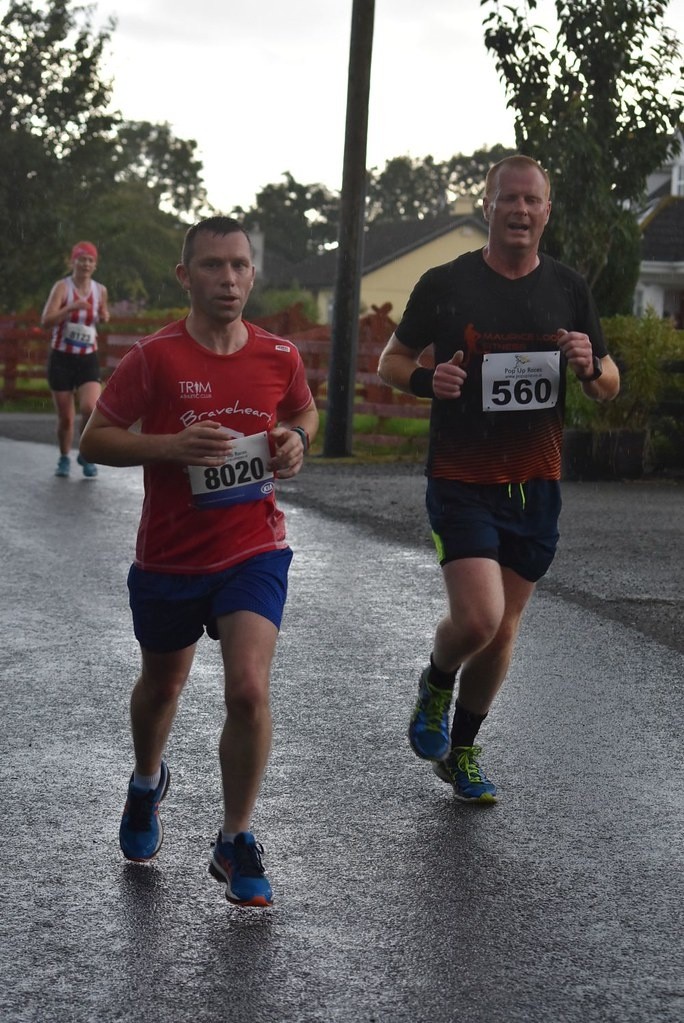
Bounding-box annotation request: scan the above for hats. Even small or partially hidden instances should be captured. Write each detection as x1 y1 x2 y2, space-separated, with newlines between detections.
70 241 97 265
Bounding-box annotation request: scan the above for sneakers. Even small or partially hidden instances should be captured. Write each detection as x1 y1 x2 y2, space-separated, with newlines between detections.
207 828 275 908
431 743 501 805
117 759 172 862
409 664 452 761
77 453 98 477
54 455 72 477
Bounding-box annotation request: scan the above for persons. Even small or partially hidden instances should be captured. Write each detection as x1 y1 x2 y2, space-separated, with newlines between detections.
39 239 110 479
375 155 620 804
78 215 320 910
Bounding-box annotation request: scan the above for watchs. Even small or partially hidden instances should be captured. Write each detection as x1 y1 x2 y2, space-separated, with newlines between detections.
291 426 310 455
576 356 603 382
